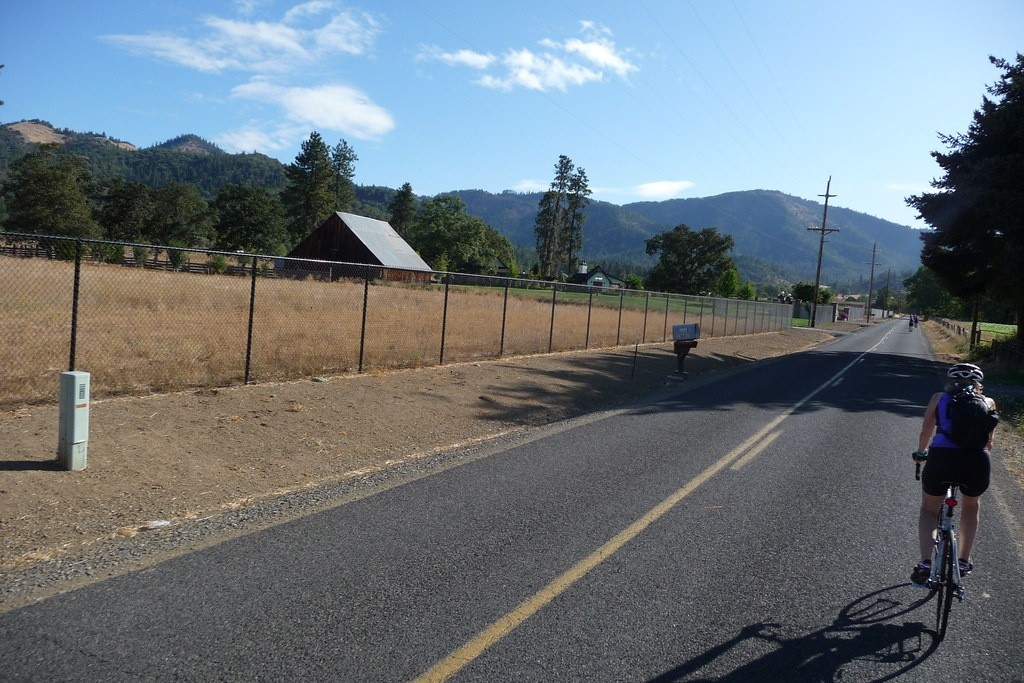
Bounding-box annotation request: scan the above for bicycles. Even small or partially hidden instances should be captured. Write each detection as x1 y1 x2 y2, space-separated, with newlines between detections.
913 455 972 642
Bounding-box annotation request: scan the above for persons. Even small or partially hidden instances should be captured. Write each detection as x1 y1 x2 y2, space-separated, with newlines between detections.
910 363 999 583
909 313 919 330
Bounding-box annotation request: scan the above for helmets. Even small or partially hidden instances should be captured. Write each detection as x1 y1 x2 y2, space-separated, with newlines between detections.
948 363 984 380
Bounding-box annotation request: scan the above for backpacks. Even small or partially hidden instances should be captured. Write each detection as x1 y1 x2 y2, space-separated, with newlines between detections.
935 384 999 452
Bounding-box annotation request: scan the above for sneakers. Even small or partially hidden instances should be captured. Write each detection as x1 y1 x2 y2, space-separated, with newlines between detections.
910 559 932 585
958 557 974 577
